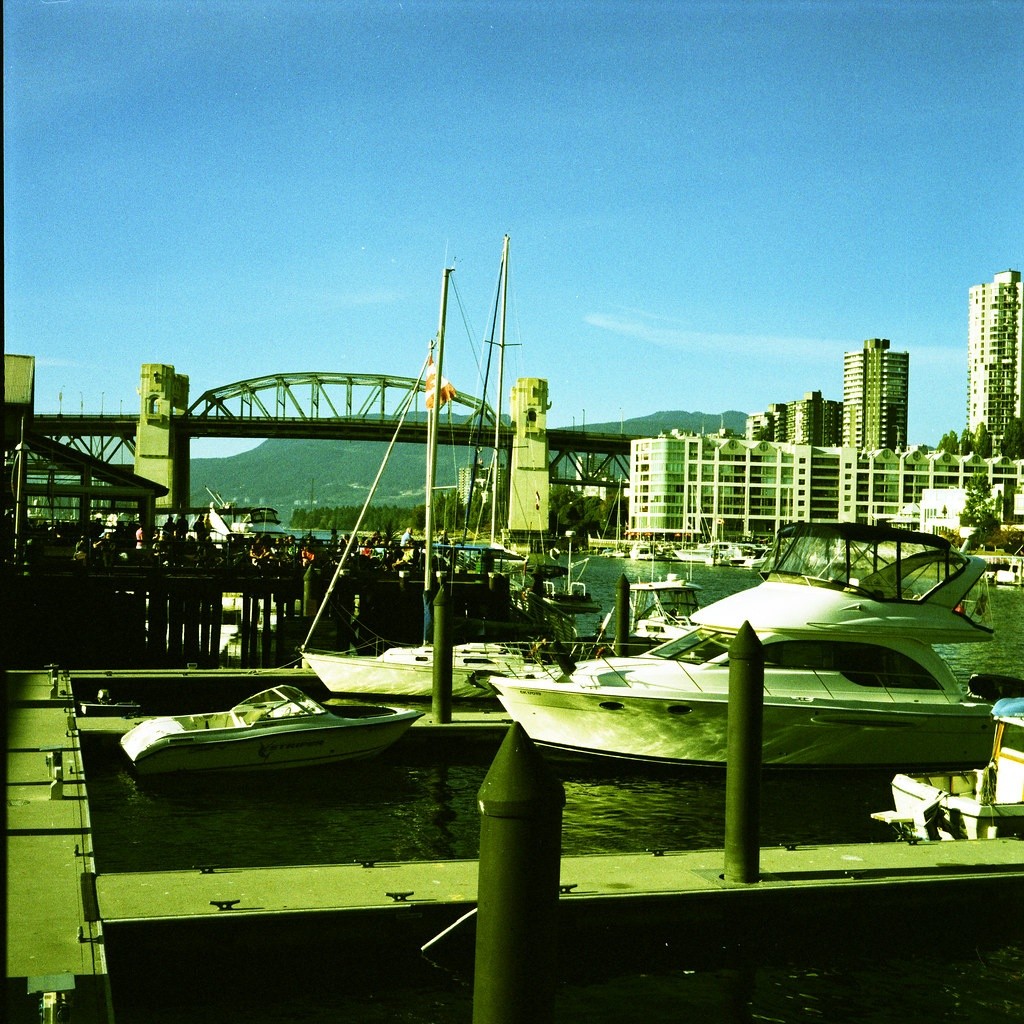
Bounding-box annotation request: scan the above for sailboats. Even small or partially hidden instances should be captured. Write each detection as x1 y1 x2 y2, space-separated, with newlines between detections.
458 233 528 561
299 236 559 698
603 473 629 558
543 530 600 614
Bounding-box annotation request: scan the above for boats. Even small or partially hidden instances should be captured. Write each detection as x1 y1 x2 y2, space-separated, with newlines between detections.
120 684 426 783
629 540 653 561
487 520 1024 769
76 690 141 717
743 548 778 568
674 540 758 567
870 697 1024 841
231 507 287 538
706 548 733 568
629 481 703 642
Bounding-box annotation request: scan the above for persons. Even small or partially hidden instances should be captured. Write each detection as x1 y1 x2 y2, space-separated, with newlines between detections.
41 513 452 574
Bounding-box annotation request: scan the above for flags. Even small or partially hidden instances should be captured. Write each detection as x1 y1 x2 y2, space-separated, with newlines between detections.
424 354 458 410
